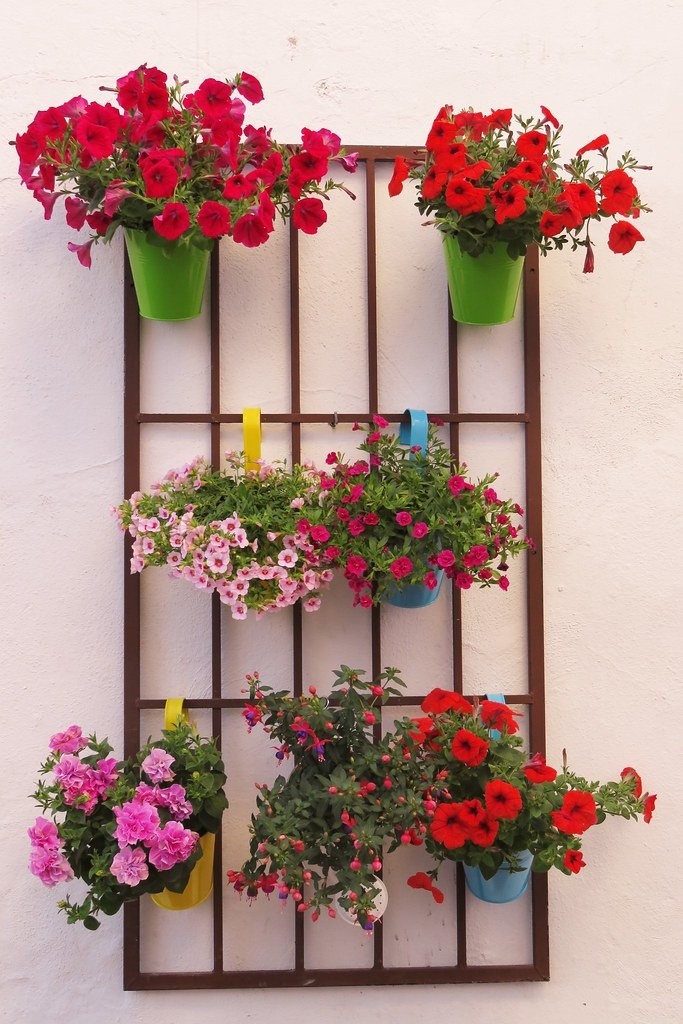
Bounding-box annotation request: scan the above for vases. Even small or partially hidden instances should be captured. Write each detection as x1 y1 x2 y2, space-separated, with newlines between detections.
370 545 446 608
443 229 524 326
145 828 217 912
122 226 203 320
470 850 533 903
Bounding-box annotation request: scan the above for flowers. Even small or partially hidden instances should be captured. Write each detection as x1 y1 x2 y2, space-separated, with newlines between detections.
232 664 452 927
404 694 660 903
12 63 355 269
388 105 649 273
117 457 335 622
306 410 539 609
24 719 229 930
